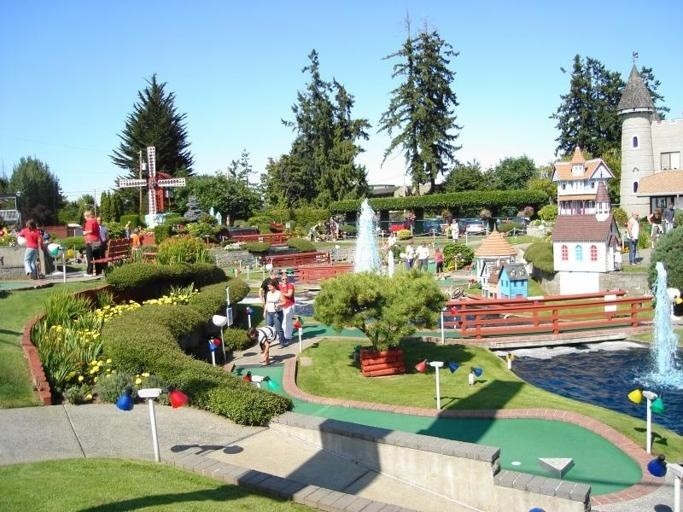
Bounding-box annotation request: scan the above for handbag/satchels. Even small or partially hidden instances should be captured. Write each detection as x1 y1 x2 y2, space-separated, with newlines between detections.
90 241 101 251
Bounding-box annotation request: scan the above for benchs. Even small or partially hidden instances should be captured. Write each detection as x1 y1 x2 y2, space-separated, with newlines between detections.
90 239 129 276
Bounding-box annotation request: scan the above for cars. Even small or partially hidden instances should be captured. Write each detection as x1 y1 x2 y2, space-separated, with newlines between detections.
343 213 529 237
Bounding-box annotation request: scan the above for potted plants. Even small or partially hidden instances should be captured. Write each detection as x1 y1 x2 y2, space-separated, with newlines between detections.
314 271 451 378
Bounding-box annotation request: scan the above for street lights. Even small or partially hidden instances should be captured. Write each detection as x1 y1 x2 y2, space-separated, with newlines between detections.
15 189 21 230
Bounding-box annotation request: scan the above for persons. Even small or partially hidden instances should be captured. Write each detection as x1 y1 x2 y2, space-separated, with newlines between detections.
35 222 44 236
96 216 109 248
131 227 141 250
627 210 640 265
416 241 430 274
449 218 458 244
262 279 288 349
663 202 675 231
124 220 132 238
275 273 294 346
647 207 665 249
386 232 396 247
43 233 59 248
81 210 104 279
405 241 415 269
435 246 445 276
258 271 279 304
245 326 277 366
18 219 43 279
